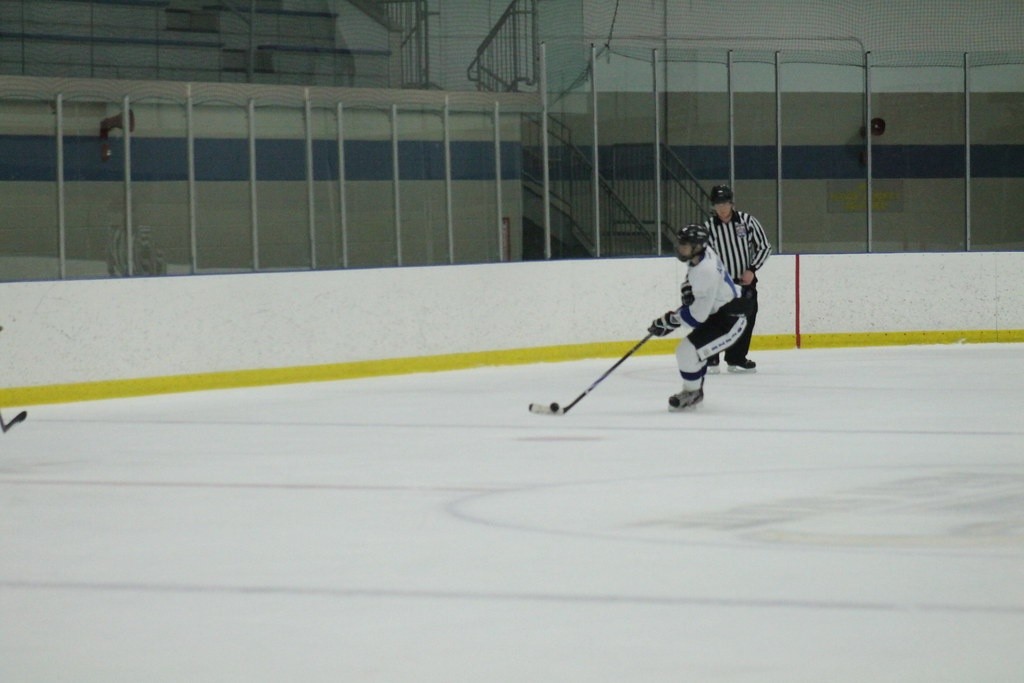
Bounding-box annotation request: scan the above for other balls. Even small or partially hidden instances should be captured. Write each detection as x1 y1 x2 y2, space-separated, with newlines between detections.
548 401 561 414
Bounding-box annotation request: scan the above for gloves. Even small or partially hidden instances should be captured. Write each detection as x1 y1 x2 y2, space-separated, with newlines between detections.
648 311 681 338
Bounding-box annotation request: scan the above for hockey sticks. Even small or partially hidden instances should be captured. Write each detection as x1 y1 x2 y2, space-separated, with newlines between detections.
525 329 656 418
0 410 28 434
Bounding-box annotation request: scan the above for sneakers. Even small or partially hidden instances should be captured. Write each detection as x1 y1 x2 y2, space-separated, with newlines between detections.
706 358 721 374
668 389 704 412
727 357 756 374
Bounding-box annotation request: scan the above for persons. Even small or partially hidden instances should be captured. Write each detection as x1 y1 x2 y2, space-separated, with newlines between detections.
647 223 748 408
700 185 772 370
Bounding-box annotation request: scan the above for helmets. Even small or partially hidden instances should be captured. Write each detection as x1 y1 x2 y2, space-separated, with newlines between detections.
677 224 709 245
711 185 732 204
